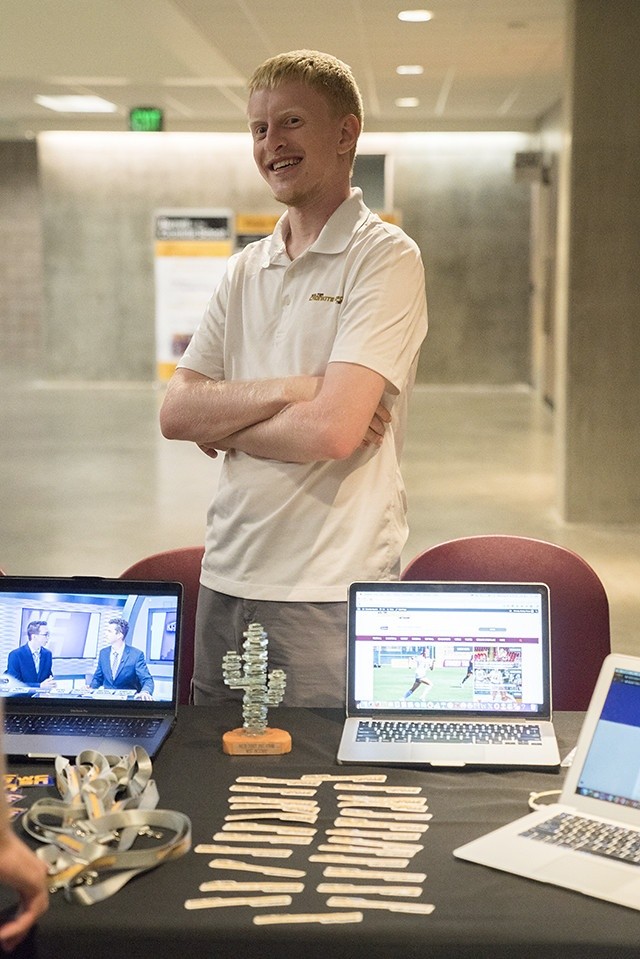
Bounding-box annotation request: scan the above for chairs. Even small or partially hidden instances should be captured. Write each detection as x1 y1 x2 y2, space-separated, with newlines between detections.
120 546 207 705
399 535 611 712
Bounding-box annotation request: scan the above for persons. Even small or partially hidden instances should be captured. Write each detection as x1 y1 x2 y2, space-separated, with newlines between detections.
3 621 56 691
0 714 50 950
89 615 155 701
158 50 430 709
460 655 473 687
400 647 435 701
474 648 522 701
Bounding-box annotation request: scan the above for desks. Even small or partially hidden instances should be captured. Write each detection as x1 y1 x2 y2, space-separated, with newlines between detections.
0 701 640 959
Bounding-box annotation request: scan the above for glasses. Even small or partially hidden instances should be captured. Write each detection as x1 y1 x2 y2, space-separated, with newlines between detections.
34 633 49 637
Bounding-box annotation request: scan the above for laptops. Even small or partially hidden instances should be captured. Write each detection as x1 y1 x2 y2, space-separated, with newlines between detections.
336 582 562 773
0 573 182 765
452 654 640 912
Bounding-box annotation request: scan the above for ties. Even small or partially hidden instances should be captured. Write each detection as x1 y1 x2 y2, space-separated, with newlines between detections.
112 652 118 681
34 651 39 663
